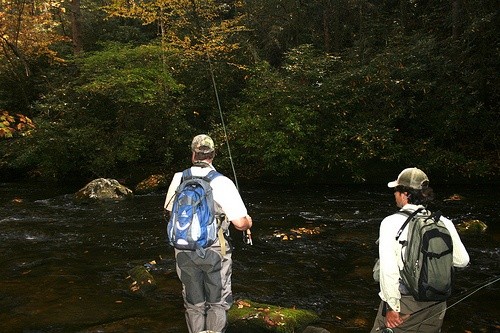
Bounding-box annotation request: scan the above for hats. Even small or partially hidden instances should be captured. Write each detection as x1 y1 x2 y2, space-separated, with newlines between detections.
187 134 215 153
387 166 429 190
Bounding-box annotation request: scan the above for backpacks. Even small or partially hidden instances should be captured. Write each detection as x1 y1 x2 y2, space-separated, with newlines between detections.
395 209 456 302
166 167 230 252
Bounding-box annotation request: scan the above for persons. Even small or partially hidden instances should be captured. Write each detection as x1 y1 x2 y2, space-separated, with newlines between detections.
163 134 253 333
372 167 469 333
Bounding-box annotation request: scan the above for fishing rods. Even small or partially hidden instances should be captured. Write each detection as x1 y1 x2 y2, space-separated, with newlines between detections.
376 277 500 332
200 27 255 245
